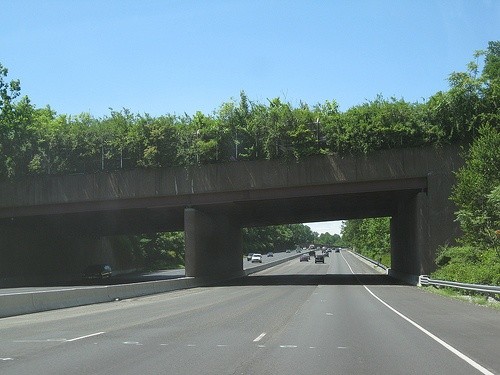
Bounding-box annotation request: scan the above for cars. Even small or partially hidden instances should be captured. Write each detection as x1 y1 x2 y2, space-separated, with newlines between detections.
286 245 339 257
81 264 112 284
267 252 274 256
251 254 263 264
247 253 253 261
300 255 308 262
315 255 324 264
